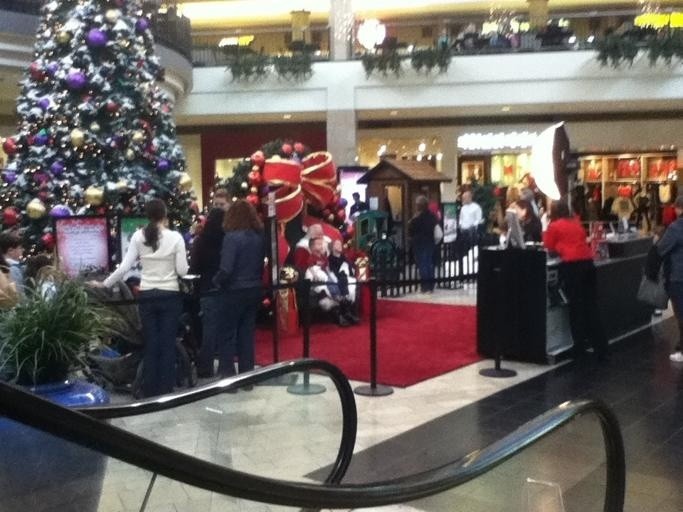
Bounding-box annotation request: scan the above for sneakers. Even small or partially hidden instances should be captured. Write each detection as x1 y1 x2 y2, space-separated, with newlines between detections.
669 352 683 362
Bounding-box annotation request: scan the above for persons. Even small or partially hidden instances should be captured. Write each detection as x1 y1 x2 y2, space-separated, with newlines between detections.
406 174 682 363
84 189 266 395
349 192 367 222
290 216 362 326
0 235 57 312
437 27 520 51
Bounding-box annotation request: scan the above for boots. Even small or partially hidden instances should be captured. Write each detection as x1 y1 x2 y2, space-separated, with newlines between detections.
330 301 359 326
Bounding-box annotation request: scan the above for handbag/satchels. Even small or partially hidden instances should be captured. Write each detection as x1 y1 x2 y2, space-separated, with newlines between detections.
433 224 443 246
636 262 669 309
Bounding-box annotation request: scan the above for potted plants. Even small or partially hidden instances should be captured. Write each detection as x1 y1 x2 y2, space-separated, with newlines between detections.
0 265 130 512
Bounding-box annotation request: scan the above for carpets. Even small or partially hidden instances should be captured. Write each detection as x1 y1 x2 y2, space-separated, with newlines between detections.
234 298 486 387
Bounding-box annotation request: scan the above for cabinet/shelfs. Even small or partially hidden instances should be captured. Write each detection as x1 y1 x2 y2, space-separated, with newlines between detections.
457 149 677 232
